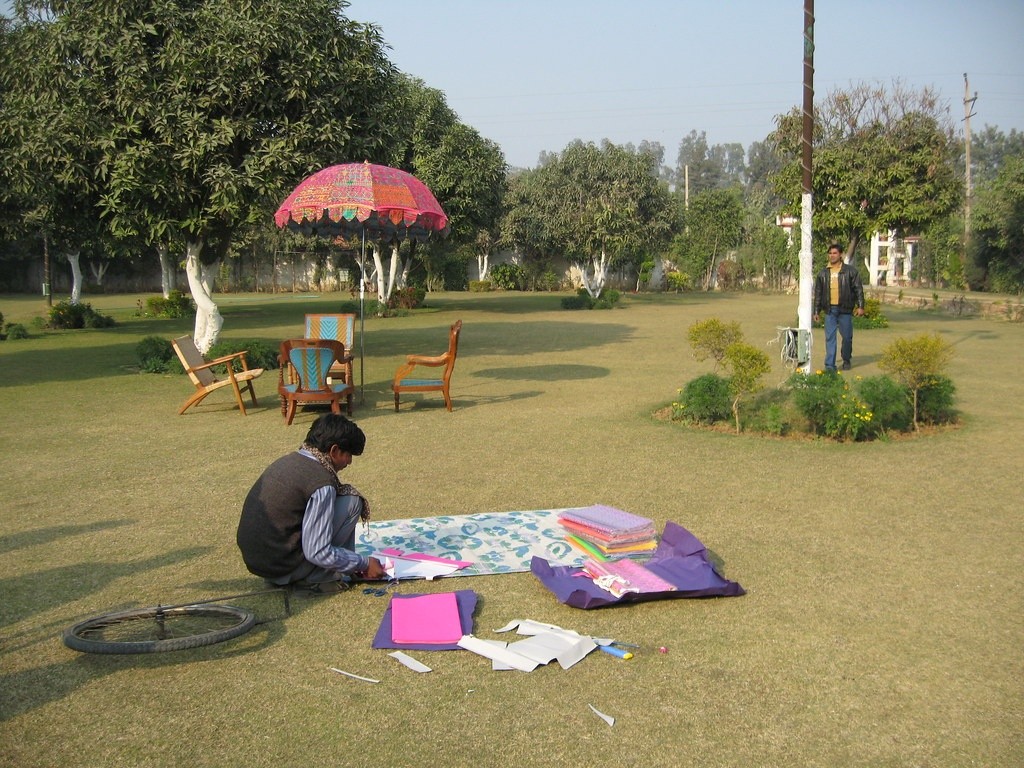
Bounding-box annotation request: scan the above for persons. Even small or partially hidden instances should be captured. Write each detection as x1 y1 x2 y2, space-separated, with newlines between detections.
813 244 864 373
237 413 387 596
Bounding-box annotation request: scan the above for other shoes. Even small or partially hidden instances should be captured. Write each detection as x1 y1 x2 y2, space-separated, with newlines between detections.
293 580 342 597
842 360 851 370
825 368 837 374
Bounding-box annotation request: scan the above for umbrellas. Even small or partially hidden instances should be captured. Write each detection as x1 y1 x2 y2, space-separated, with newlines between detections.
274 159 448 406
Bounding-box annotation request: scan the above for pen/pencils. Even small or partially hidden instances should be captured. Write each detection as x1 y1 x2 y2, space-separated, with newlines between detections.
612 641 639 647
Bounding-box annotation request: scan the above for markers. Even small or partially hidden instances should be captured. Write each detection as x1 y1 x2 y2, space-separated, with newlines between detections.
598 645 633 661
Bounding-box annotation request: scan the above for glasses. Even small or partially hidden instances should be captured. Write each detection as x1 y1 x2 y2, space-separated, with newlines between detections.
828 252 837 254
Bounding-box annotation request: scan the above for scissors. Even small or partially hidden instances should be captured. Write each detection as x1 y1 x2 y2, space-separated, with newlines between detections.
363 582 395 597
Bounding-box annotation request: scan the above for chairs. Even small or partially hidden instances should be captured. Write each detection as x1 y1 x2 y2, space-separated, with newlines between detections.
171 334 263 416
287 312 357 384
282 338 353 426
393 319 463 412
